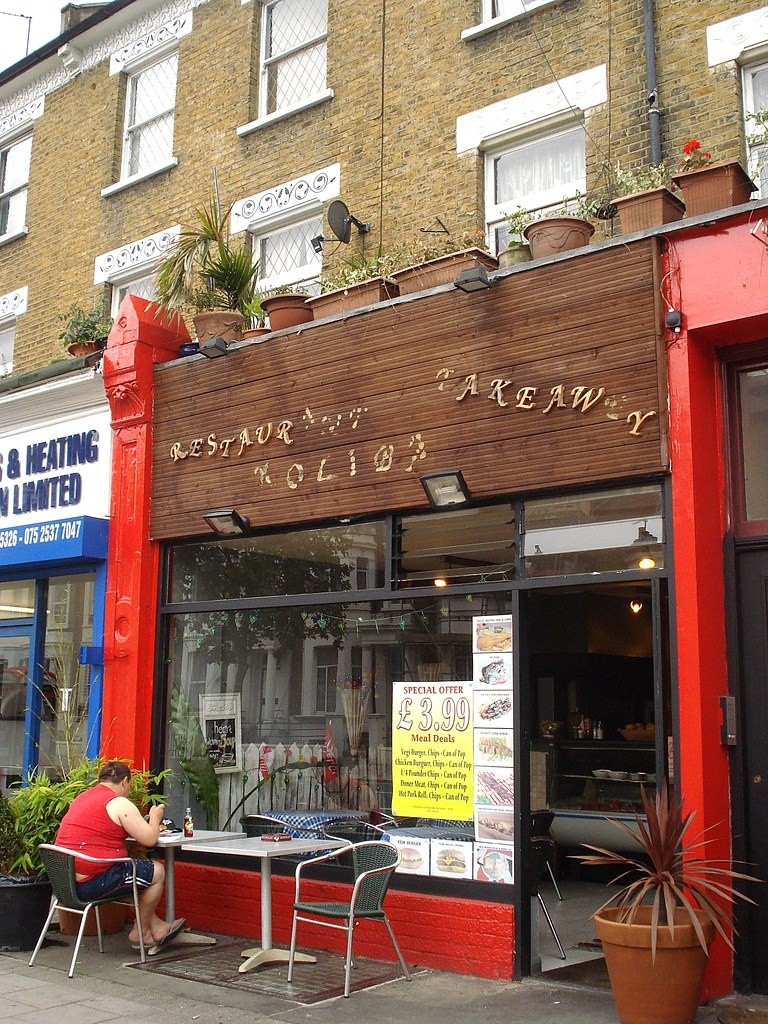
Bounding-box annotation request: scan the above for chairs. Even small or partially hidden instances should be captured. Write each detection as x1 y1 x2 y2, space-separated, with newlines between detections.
523 808 572 961
28 843 148 977
228 805 413 1000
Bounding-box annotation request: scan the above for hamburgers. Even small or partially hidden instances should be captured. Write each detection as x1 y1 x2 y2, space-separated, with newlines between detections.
437 850 466 872
399 848 423 868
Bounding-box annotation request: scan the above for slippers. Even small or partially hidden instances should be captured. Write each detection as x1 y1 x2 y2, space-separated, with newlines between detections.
132 937 168 949
160 917 186 945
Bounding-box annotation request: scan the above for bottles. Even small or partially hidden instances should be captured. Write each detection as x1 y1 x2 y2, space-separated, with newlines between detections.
572 714 606 740
183 807 193 837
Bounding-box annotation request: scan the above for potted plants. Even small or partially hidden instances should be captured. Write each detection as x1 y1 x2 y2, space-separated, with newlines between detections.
12 753 174 937
243 299 273 340
191 250 263 346
0 788 53 953
497 244 534 270
584 779 764 1024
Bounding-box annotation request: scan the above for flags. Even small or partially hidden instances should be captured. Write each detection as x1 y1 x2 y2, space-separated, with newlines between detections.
324 726 338 784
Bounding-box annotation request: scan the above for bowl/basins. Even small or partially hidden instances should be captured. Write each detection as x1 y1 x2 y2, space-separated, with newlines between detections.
607 771 628 780
592 769 610 778
646 774 656 781
627 773 646 781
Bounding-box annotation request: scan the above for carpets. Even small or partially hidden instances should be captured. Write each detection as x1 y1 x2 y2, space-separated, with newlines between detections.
121 942 428 1008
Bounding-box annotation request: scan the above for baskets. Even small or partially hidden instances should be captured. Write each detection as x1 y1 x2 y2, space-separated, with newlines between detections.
617 728 655 741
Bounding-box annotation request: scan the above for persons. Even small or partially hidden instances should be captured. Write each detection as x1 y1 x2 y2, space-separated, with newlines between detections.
52 761 187 949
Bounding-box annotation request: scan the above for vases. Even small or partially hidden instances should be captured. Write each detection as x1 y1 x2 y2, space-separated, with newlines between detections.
67 341 93 358
258 290 313 330
671 158 759 221
178 342 199 357
95 337 109 350
610 186 684 236
522 217 594 260
393 250 493 299
305 275 403 320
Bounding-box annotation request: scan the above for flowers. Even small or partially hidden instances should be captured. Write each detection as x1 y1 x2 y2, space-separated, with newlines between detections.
54 293 117 346
257 282 319 297
681 140 730 174
618 161 685 198
505 191 601 237
315 254 395 297
391 229 495 279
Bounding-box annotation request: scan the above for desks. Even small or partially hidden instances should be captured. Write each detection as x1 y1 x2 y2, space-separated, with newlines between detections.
117 828 246 954
241 809 370 860
415 819 473 829
180 837 347 974
381 827 476 847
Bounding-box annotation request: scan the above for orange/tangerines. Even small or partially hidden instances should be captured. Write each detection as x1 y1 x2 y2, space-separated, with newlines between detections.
624 722 654 730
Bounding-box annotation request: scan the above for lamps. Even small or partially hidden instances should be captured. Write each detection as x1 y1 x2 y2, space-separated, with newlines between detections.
203 510 251 540
421 472 472 506
638 545 657 569
630 590 643 612
197 336 229 361
453 266 496 293
